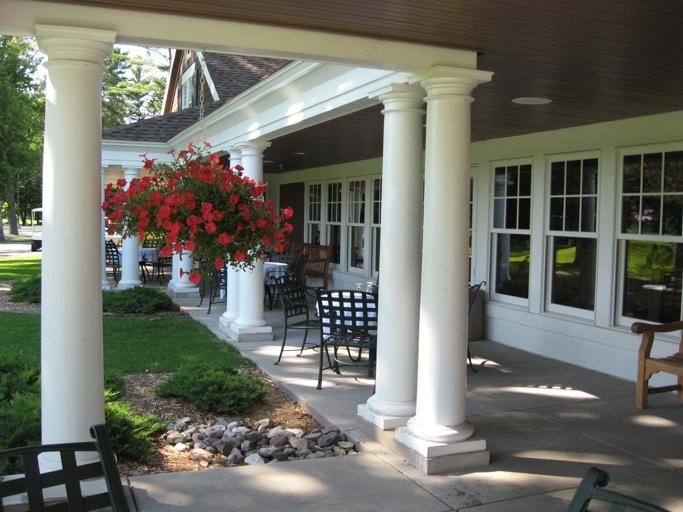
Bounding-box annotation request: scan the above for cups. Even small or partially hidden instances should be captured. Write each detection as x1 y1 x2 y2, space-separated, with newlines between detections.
355 282 362 292
366 281 374 293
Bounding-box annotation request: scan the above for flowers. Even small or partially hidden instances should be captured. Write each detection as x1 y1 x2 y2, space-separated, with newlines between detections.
101 144 295 283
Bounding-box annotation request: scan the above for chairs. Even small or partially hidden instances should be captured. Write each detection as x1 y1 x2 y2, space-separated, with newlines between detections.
468 280 488 372
275 274 332 367
566 466 672 512
630 322 683 410
105 236 331 311
0 424 133 512
317 290 377 390
